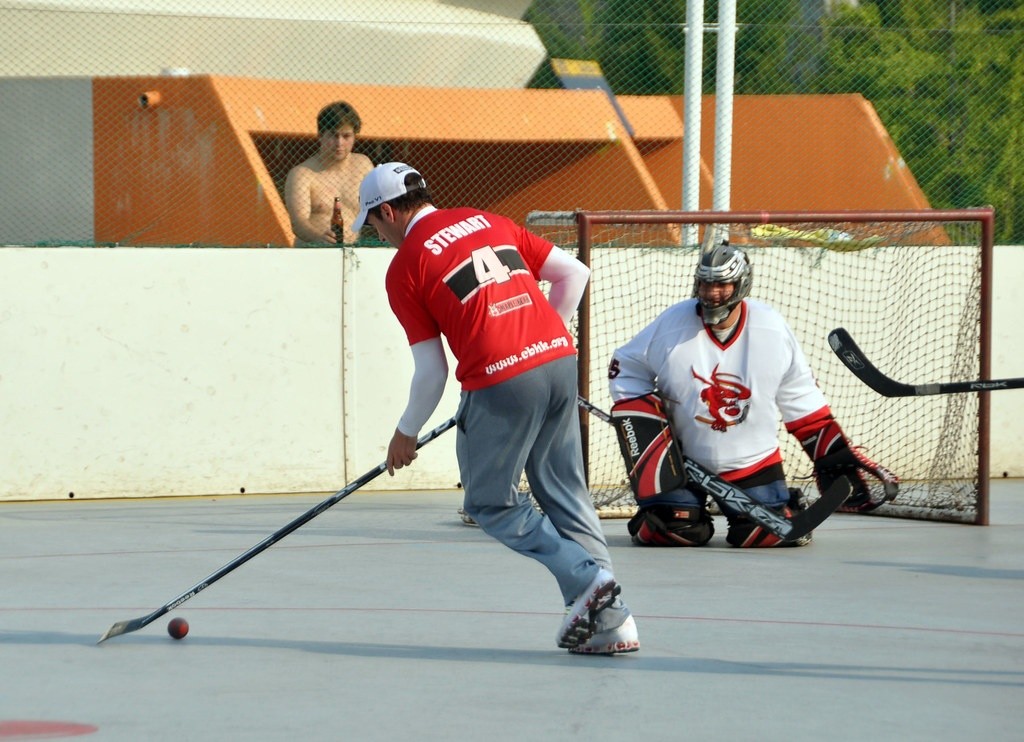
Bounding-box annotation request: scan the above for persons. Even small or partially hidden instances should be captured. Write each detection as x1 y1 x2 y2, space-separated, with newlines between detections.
284 101 375 245
351 162 641 654
608 240 870 548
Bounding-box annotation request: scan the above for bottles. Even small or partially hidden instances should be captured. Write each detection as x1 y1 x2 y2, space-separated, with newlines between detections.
331 197 344 243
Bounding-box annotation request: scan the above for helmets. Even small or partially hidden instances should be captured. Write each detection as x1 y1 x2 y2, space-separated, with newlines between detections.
690 239 755 325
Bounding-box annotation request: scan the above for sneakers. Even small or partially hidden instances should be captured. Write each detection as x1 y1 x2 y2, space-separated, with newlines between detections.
556 567 621 648
568 614 640 653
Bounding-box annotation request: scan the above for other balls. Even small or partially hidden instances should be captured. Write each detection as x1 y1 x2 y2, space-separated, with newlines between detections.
167 617 190 640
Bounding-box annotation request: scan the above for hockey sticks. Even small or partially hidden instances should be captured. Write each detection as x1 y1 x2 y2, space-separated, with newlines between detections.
577 395 853 542
827 327 1024 398
96 415 458 646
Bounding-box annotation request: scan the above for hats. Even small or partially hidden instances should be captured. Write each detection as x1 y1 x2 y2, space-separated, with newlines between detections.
351 162 426 232
